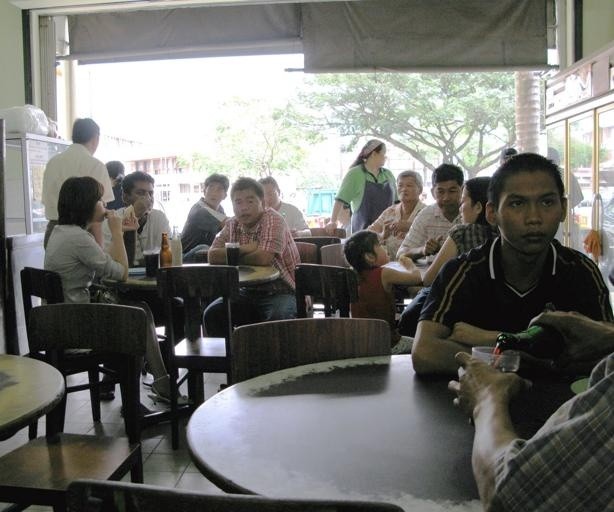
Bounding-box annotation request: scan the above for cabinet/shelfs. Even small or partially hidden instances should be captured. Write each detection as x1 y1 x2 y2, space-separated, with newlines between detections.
539 41 614 299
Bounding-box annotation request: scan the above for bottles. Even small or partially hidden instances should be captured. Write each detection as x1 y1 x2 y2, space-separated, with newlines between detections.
169 225 183 266
159 231 172 268
494 320 574 361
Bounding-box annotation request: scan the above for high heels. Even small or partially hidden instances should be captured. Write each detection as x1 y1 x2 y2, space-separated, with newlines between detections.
147 373 195 405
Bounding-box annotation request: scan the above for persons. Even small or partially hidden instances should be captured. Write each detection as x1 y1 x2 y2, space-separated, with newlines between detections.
323 139 517 355
205 178 313 392
42 117 116 252
410 153 614 383
42 176 190 407
548 146 585 210
256 175 308 232
96 171 186 401
106 160 128 209
179 171 230 263
447 271 614 512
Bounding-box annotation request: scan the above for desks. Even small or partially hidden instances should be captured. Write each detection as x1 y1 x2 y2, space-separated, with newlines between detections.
0 353 65 442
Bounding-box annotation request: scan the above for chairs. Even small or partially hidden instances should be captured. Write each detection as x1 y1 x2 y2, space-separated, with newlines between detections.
90 227 360 451
19 266 101 439
0 303 149 512
62 477 403 512
231 318 392 385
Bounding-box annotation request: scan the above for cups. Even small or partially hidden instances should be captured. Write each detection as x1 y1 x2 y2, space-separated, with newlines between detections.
467 346 522 426
142 248 160 278
225 242 242 266
